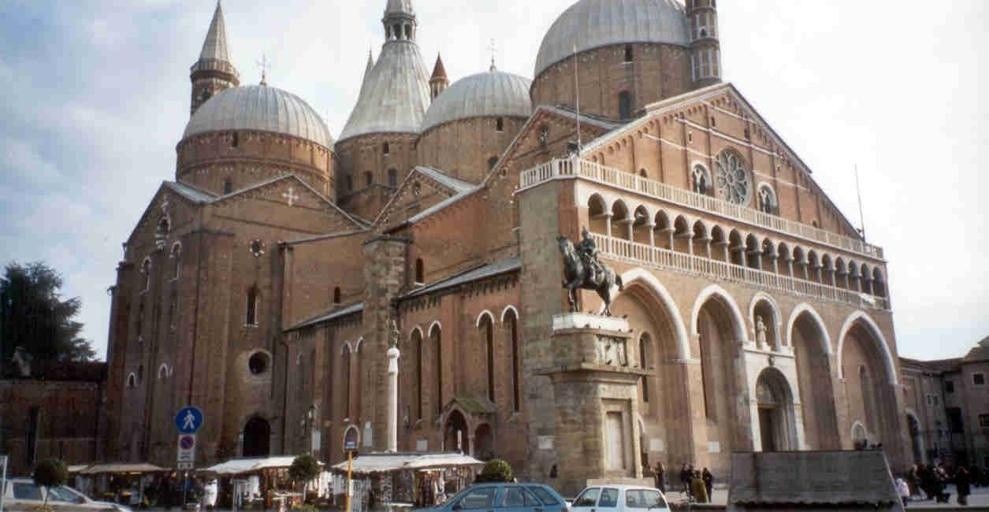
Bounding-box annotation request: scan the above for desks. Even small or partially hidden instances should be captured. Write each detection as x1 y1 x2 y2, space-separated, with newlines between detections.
384 502 414 512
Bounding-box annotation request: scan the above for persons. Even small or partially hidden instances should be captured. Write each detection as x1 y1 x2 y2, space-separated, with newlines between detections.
642 463 655 477
910 460 972 505
678 461 690 494
656 461 666 494
756 314 768 348
702 467 713 503
574 226 602 287
63 471 220 512
685 463 697 495
896 478 911 507
691 470 709 504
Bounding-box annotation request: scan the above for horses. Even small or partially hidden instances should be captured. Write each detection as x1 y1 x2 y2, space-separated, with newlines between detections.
555 233 626 315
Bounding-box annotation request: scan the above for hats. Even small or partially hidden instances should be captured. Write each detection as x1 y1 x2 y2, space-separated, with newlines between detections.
694 470 703 474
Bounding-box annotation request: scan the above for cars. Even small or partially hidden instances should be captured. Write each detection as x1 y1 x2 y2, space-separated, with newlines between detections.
570 486 671 511
0 476 133 512
410 483 571 512
